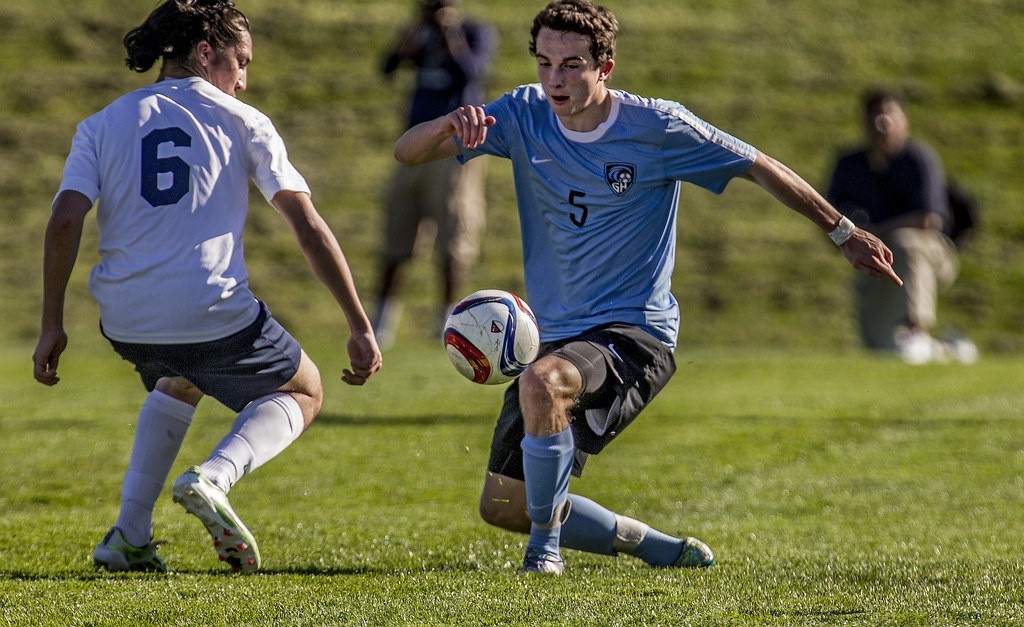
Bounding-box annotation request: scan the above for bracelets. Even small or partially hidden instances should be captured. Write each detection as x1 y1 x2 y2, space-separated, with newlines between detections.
827 215 856 246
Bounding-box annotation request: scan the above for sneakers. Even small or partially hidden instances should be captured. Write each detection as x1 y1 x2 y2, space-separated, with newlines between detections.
522 549 563 577
170 466 262 575
93 527 167 574
673 537 715 570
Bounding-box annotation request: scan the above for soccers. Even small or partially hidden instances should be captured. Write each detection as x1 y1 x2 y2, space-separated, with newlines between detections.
440 287 540 387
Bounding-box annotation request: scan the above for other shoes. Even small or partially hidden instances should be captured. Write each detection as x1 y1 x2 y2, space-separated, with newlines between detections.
898 326 931 365
952 338 977 365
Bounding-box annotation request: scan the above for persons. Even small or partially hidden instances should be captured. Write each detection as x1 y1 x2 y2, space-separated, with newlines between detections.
395 0 903 575
825 92 981 365
33 0 384 574
357 0 501 354
